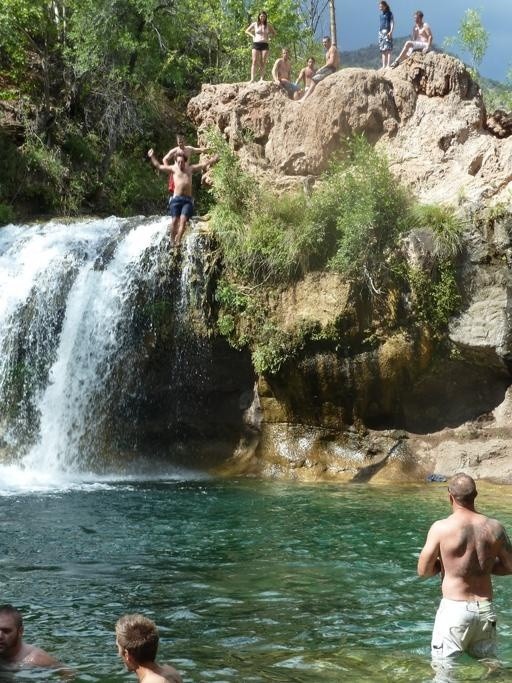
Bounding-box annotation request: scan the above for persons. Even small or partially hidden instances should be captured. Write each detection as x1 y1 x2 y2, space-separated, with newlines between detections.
390 11 432 67
0 603 77 683
244 11 278 85
294 36 340 104
270 47 301 101
162 131 216 192
417 474 512 683
377 1 394 72
114 613 184 683
146 148 220 247
294 55 316 96
